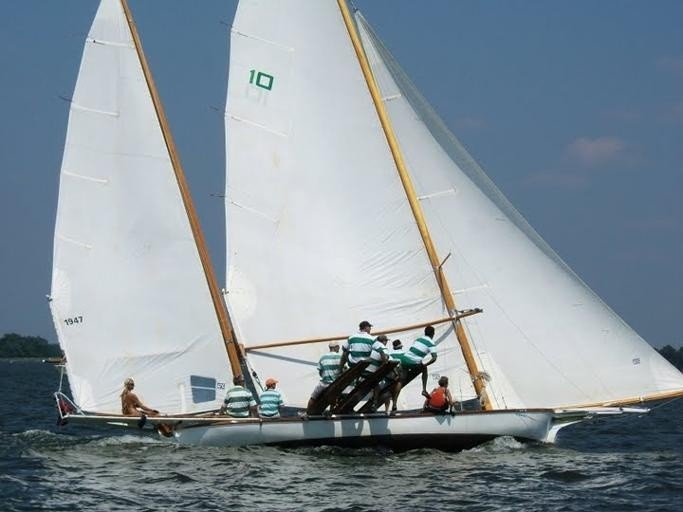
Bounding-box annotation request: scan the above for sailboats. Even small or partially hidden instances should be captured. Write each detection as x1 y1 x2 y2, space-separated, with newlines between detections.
50 0 683 450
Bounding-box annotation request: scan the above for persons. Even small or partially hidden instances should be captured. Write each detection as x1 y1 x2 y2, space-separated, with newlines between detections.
402 325 438 399
385 338 422 417
120 378 170 434
334 320 390 377
257 377 285 418
423 375 454 414
354 335 401 412
300 342 343 420
219 374 257 418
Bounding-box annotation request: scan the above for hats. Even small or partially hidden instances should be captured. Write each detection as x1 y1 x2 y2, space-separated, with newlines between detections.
359 320 372 329
392 339 400 346
328 341 340 347
265 378 278 385
378 336 389 340
